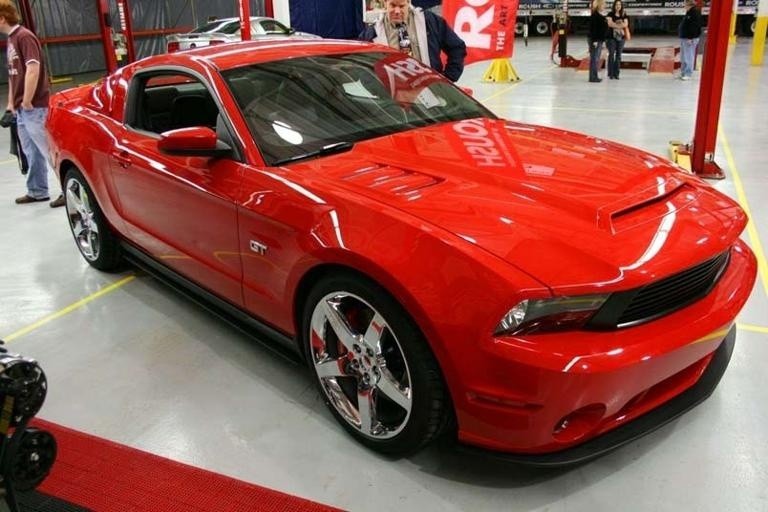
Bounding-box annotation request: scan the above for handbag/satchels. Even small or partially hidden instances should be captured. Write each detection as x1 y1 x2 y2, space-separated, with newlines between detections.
625 28 631 42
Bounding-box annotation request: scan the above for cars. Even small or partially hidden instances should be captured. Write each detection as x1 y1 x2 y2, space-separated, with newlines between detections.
44 15 759 469
165 16 324 54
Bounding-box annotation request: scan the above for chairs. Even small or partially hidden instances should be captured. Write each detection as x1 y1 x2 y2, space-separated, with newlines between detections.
142 75 286 164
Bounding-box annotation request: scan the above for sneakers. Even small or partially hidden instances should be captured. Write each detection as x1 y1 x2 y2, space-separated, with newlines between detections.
15 195 65 208
589 76 618 82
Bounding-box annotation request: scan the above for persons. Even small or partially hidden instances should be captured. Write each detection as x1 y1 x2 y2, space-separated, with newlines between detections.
0 1 66 208
587 0 631 82
678 1 702 80
357 0 466 82
549 8 570 60
